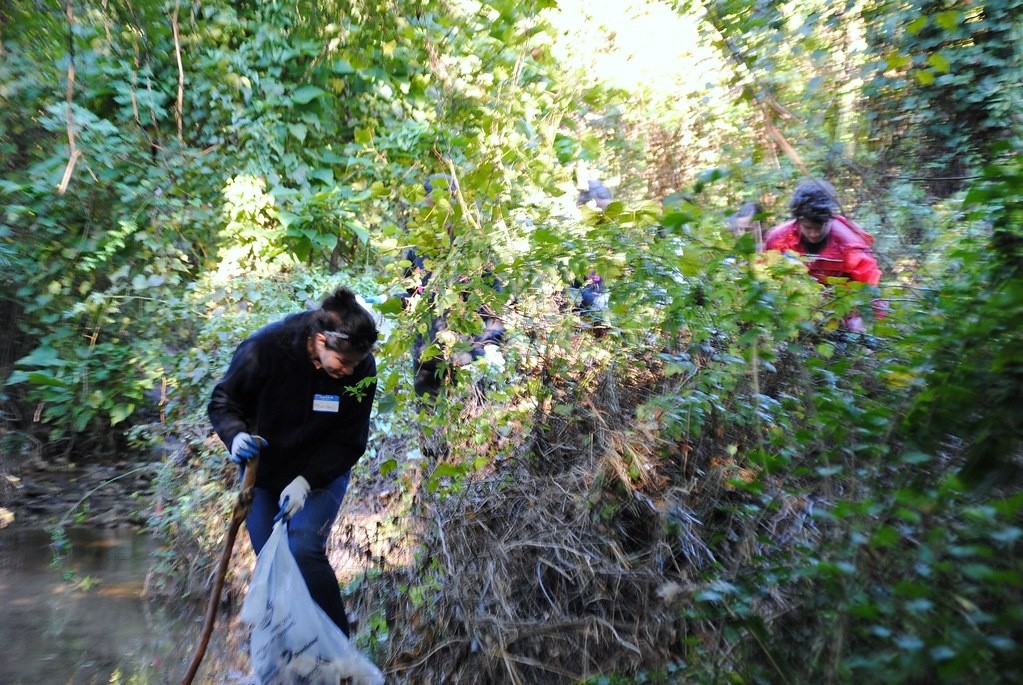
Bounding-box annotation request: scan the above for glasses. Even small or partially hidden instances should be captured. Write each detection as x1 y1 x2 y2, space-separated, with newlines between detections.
324 336 375 354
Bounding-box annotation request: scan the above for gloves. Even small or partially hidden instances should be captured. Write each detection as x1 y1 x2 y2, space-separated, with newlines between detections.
231 431 267 466
272 476 313 526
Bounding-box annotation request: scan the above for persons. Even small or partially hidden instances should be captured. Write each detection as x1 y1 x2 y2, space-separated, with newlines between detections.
208 287 379 685
399 174 612 507
764 180 892 329
